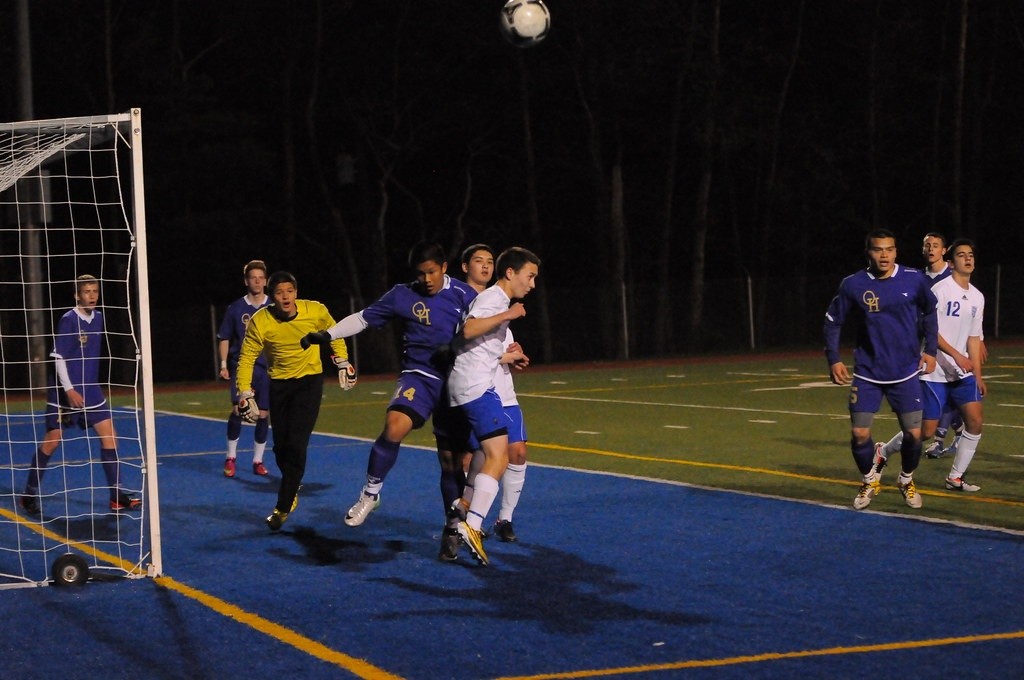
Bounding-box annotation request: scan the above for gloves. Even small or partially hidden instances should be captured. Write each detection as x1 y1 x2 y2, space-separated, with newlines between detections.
338 362 357 391
238 391 260 423
301 329 332 350
428 344 450 369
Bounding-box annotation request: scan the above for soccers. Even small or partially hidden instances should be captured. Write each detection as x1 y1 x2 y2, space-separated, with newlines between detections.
499 0 552 49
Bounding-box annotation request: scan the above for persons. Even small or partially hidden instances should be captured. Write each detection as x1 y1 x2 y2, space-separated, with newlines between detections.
921 233 987 459
461 245 534 542
445 247 541 568
216 260 274 477
873 238 988 493
235 274 357 531
823 229 939 509
300 240 523 561
20 275 141 518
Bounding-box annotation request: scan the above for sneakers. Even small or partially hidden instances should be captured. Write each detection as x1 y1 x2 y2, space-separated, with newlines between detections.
110 494 143 512
873 441 887 482
457 521 490 566
924 443 945 459
20 494 45 521
853 480 881 510
439 527 458 562
493 519 517 542
943 439 958 453
252 461 268 475
343 485 380 527
224 457 237 477
451 499 488 538
897 475 922 508
946 475 981 491
265 493 297 531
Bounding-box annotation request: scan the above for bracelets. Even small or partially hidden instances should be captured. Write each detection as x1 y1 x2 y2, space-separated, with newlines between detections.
220 361 226 369
